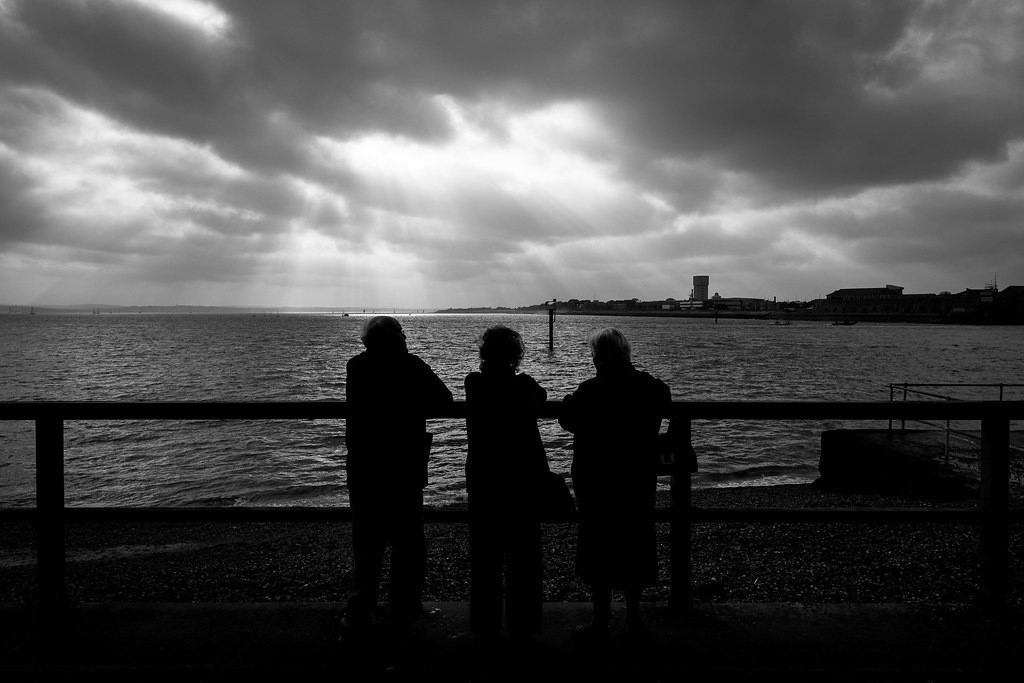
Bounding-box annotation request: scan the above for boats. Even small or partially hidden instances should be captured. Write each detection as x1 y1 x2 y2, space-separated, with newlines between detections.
769 321 793 325
831 320 859 326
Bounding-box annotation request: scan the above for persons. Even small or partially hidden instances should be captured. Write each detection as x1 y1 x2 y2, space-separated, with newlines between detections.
334 317 672 648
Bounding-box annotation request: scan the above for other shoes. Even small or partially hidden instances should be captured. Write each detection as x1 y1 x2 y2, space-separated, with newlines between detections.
510 634 534 648
338 612 374 632
570 622 609 641
475 631 501 644
388 621 428 644
623 623 645 638
